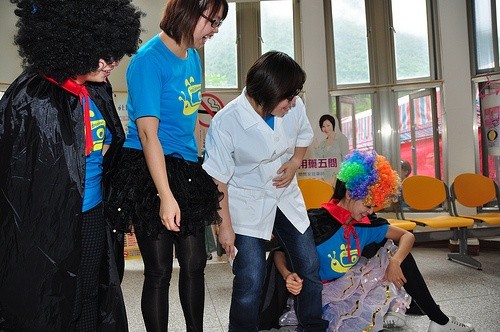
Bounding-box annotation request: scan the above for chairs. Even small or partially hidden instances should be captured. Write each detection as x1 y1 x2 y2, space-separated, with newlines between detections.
299 178 335 210
396 176 484 270
450 172 500 241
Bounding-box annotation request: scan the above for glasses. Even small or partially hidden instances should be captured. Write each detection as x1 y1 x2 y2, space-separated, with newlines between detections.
287 86 303 101
200 13 222 29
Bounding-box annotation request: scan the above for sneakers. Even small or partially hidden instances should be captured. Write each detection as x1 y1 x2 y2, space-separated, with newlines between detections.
383 311 407 328
427 315 474 332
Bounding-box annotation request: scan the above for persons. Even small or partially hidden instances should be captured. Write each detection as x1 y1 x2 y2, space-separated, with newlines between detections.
399 159 412 183
0 0 142 332
272 148 475 332
201 51 331 332
121 0 232 332
312 114 349 187
